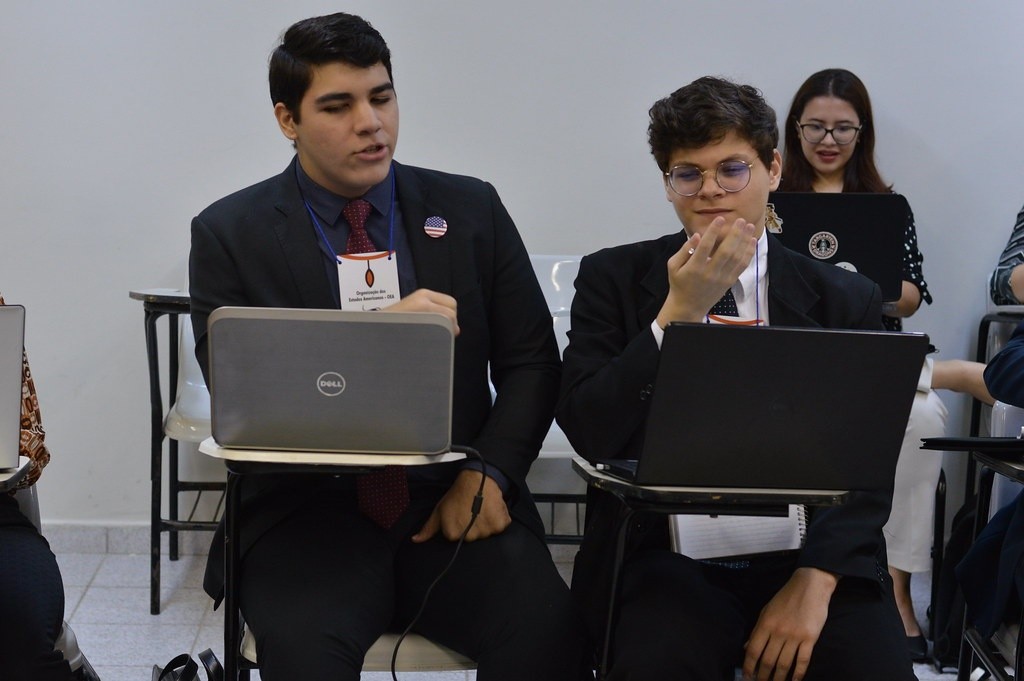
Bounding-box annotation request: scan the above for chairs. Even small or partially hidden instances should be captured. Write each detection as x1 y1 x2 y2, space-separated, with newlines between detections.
146 253 1024 681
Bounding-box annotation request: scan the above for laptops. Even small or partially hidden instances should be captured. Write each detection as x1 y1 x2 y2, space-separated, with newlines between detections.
0 305 25 470
208 306 454 456
591 322 930 492
765 192 904 305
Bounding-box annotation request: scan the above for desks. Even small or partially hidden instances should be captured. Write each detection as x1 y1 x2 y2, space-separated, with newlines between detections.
571 456 874 518
0 456 30 493
129 288 593 616
990 304 1024 324
198 436 466 475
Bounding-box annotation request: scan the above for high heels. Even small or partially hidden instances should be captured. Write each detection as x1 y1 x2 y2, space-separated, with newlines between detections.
906 621 927 662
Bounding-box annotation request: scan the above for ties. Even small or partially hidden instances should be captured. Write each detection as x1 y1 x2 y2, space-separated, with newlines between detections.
706 288 739 318
342 200 410 529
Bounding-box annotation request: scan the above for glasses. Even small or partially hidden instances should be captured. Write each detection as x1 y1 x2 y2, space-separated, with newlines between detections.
664 157 766 196
790 119 866 146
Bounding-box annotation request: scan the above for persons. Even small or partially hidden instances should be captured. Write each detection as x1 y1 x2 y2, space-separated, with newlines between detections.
774 67 1024 664
0 292 88 681
188 12 583 681
559 74 911 681
